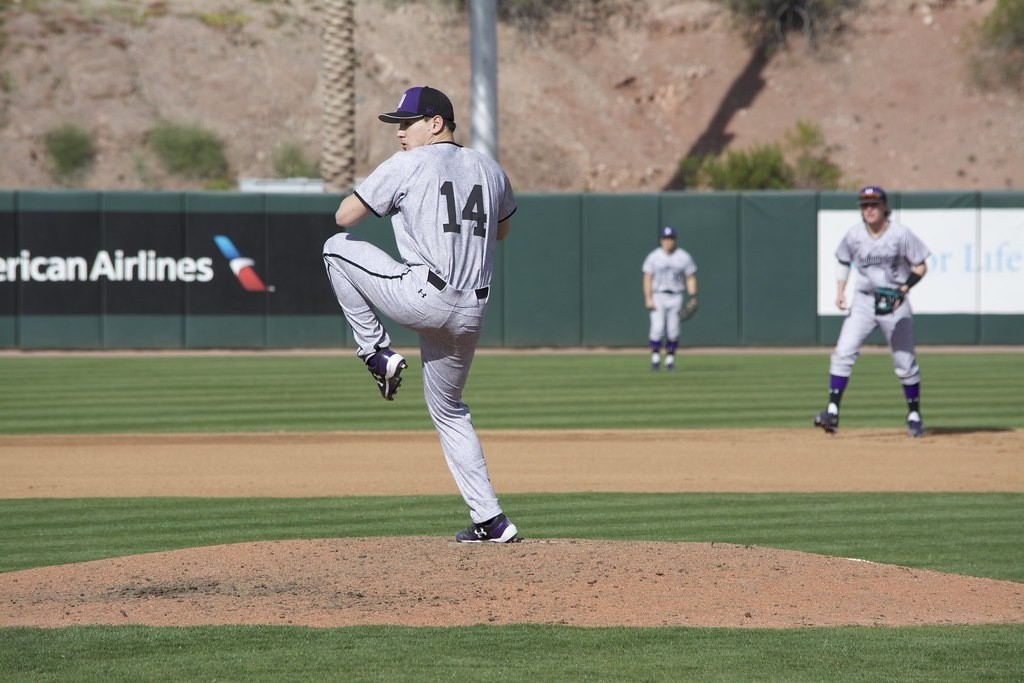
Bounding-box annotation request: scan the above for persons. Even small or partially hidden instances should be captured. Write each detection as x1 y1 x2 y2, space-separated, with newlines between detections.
813 187 934 435
322 87 517 543
642 227 700 371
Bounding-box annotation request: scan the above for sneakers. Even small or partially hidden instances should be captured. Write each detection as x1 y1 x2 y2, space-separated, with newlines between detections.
906 411 924 437
368 348 408 401
456 514 518 544
815 408 840 433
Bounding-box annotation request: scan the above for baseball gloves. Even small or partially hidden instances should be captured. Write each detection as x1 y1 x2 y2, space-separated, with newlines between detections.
872 287 905 315
677 295 699 322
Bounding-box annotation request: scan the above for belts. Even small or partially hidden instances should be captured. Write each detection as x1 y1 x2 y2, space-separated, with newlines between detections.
427 270 488 299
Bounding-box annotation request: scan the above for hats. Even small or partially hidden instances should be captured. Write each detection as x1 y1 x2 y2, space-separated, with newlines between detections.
661 226 676 237
378 86 454 122
858 187 886 206
